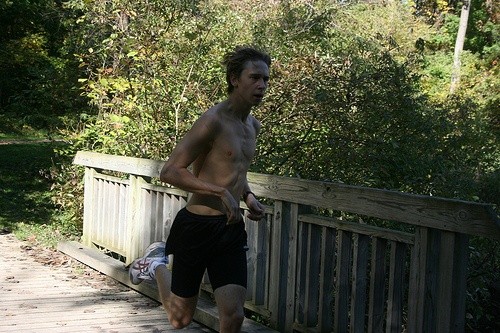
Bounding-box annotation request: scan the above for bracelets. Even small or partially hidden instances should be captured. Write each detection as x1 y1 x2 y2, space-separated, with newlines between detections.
241 191 255 202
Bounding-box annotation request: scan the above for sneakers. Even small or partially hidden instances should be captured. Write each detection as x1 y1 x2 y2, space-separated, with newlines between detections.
129 241 169 285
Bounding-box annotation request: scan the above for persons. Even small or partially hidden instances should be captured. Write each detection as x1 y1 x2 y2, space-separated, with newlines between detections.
129 44 273 333
